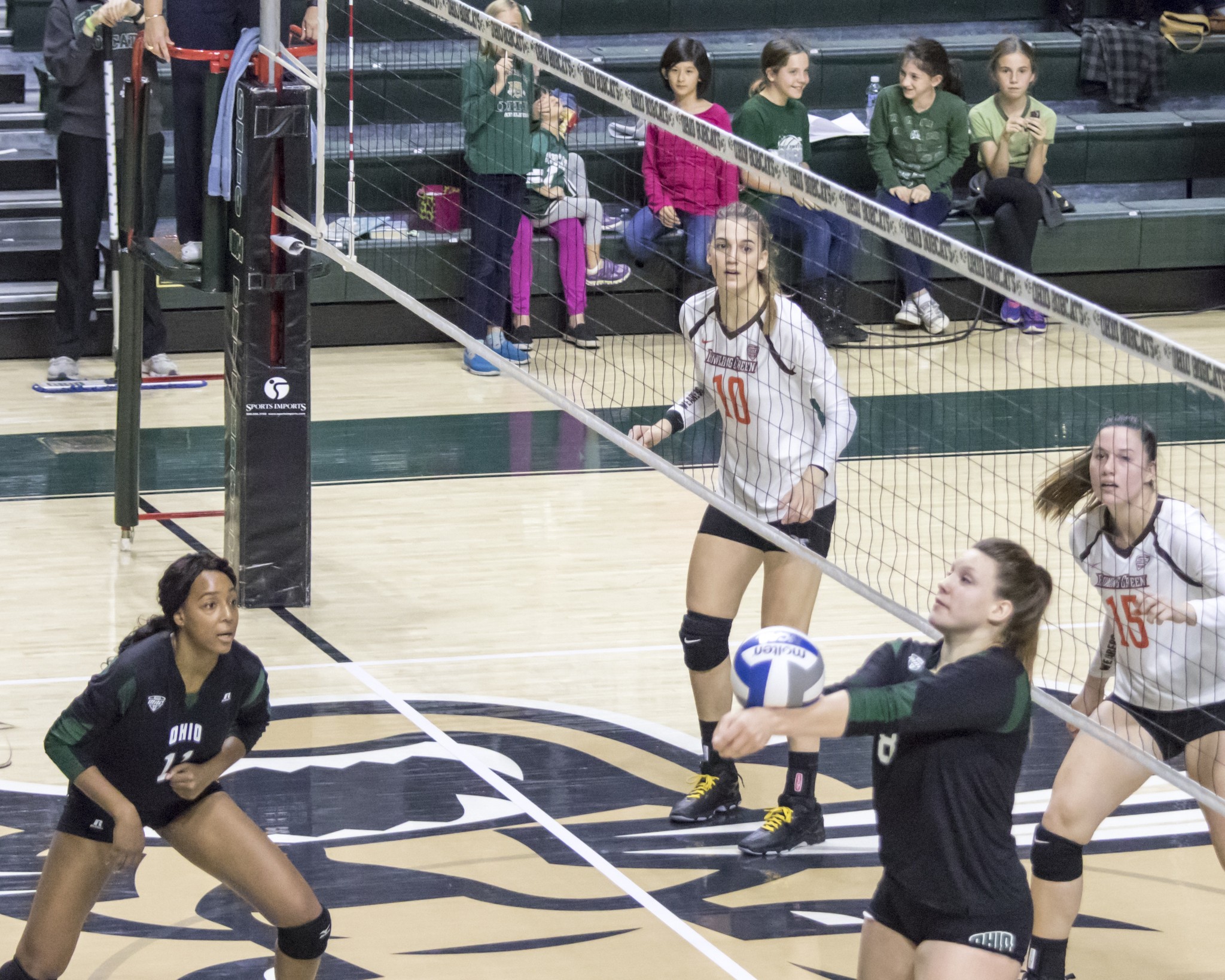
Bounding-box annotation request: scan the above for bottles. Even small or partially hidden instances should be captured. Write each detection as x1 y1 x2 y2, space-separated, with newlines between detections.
866 76 883 130
620 208 631 235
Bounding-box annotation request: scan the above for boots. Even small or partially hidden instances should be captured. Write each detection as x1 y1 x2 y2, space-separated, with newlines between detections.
797 277 848 345
826 273 868 342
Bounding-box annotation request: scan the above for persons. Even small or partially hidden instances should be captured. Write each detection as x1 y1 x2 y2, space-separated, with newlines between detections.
42 0 1059 384
626 201 860 856
0 553 333 980
711 536 1050 980
1018 417 1225 980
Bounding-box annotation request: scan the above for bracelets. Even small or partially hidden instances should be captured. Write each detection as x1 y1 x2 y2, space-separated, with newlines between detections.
146 14 163 20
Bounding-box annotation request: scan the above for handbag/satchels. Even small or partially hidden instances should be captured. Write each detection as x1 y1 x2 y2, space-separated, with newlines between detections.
1158 10 1210 54
416 184 460 233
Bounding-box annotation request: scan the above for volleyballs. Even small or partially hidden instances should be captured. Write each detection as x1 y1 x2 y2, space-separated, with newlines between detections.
731 628 824 710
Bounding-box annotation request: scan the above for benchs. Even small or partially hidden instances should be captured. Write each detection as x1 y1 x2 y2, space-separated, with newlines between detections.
0 33 1225 345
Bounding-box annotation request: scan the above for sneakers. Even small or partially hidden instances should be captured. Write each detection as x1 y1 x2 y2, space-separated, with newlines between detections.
601 213 622 230
738 794 825 855
918 297 949 334
669 760 744 822
1021 305 1047 334
1000 298 1020 324
47 356 79 381
895 297 921 326
141 353 178 377
585 255 631 286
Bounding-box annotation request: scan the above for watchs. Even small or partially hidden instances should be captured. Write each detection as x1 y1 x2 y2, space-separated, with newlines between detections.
129 3 144 21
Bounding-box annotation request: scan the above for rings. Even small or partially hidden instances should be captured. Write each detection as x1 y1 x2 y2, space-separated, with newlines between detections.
148 45 154 50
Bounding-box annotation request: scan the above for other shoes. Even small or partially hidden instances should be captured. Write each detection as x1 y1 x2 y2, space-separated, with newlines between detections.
563 323 602 348
484 339 532 364
510 323 534 350
181 241 204 263
460 348 500 376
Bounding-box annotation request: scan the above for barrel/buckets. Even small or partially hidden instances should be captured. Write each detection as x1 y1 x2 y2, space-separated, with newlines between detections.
417 185 461 232
